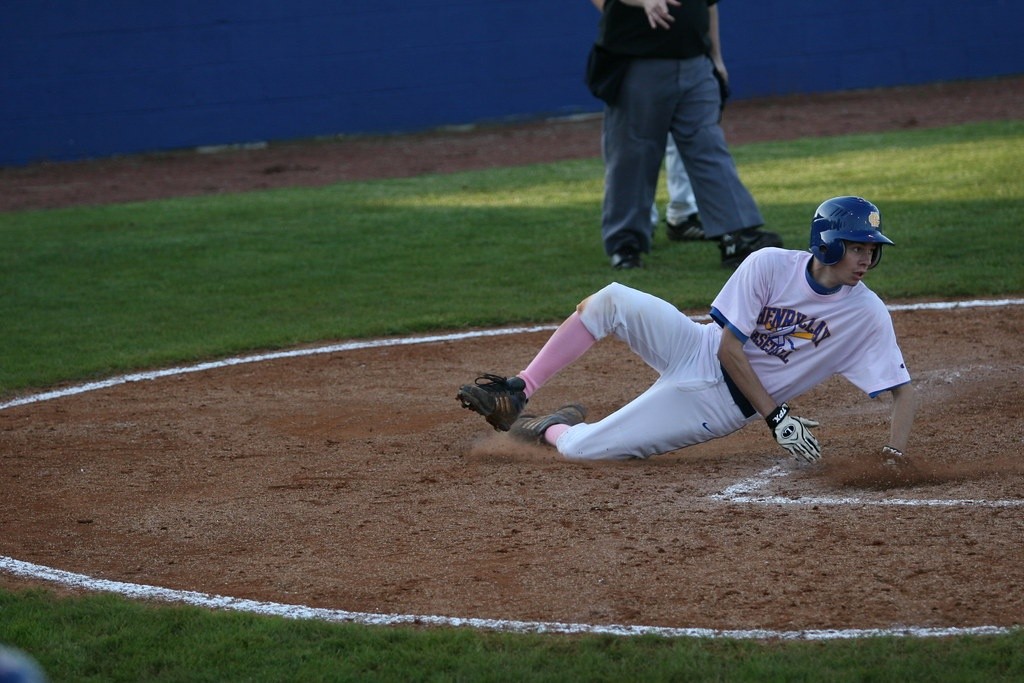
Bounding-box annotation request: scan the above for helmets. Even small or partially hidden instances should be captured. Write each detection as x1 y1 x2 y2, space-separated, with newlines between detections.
810 196 896 269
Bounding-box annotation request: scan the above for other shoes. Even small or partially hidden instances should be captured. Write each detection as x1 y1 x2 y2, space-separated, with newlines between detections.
721 228 781 265
611 247 643 270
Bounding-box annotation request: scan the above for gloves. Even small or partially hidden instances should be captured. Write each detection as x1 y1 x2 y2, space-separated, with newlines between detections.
765 402 822 465
880 446 903 478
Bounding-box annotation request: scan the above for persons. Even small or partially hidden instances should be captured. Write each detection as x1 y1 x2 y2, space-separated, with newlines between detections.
586 0 785 268
647 133 719 242
455 196 916 473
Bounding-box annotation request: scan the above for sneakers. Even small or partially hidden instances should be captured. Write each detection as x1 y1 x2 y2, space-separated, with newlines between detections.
510 402 585 447
668 214 707 240
459 373 529 432
